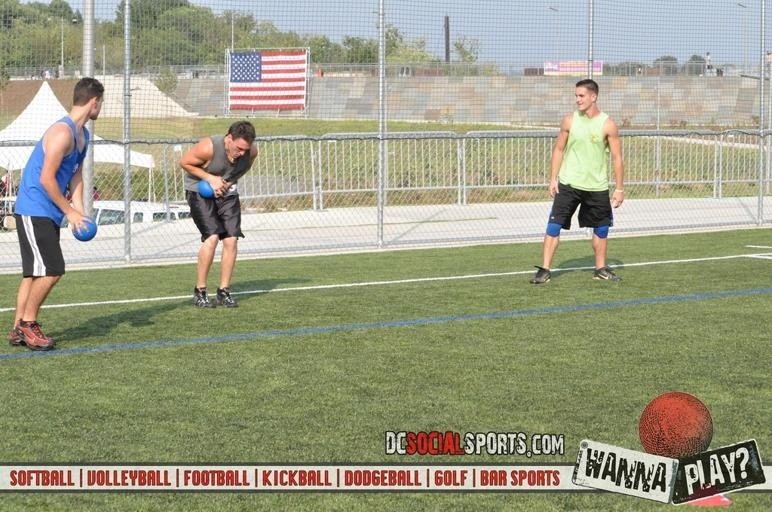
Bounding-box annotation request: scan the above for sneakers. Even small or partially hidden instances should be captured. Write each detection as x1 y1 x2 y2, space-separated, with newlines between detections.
530 265 552 284
7 319 55 351
592 266 622 282
217 287 238 307
193 286 216 307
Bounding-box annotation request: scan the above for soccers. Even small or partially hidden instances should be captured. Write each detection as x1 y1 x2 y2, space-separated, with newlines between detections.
198 179 214 199
639 393 714 458
72 221 96 241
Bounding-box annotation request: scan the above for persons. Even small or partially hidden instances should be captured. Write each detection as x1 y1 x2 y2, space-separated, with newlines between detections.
178 120 259 308
0 170 101 232
705 51 715 76
7 77 105 351
529 79 624 285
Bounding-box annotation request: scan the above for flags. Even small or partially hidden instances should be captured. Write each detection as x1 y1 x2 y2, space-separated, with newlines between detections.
228 52 307 110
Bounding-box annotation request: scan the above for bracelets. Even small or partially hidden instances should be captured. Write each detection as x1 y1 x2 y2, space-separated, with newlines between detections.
614 189 624 193
